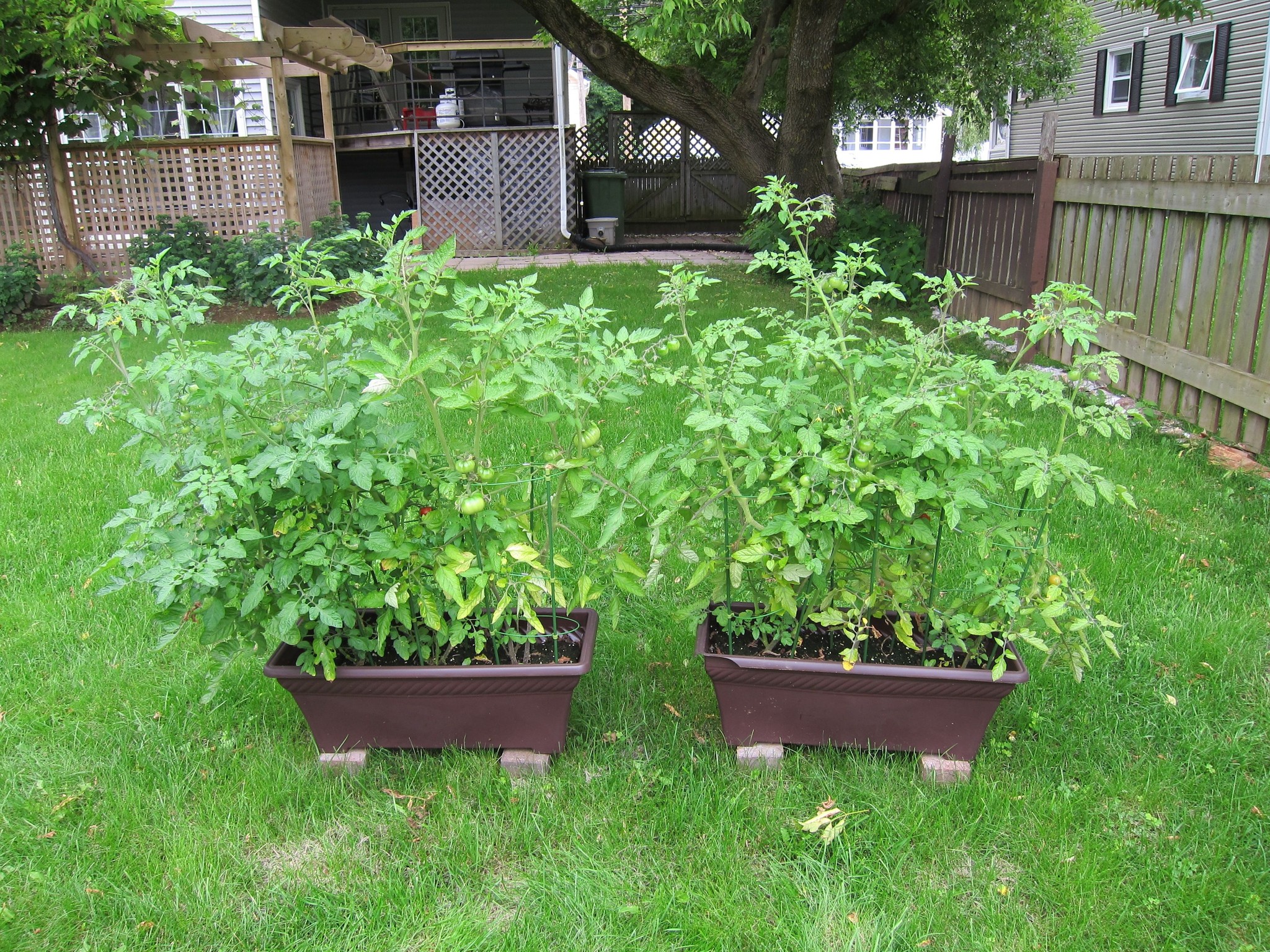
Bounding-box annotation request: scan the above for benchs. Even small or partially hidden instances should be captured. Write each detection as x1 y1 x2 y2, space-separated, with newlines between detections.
523 89 554 126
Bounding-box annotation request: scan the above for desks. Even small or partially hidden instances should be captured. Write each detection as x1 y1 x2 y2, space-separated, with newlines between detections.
402 107 436 130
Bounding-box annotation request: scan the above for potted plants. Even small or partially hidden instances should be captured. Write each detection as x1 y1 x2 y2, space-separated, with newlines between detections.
49 175 1159 761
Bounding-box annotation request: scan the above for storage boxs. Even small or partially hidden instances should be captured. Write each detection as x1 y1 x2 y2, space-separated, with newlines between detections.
585 217 619 247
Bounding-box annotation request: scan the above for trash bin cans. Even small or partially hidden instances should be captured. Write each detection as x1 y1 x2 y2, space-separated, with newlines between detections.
576 167 627 243
585 217 618 245
